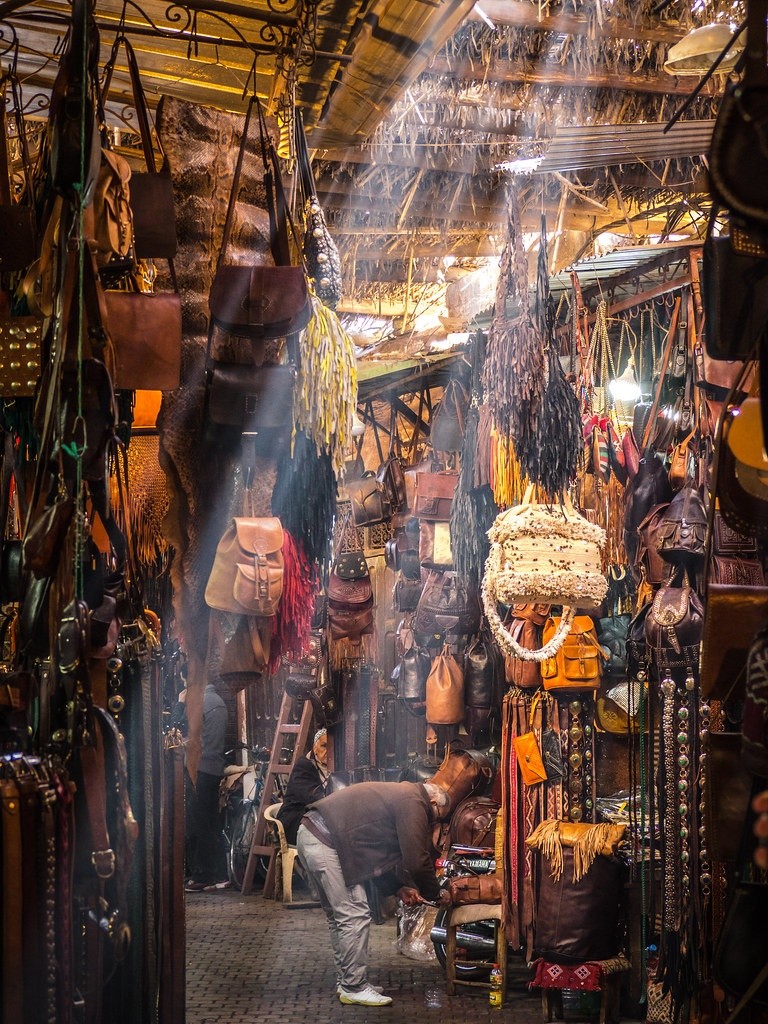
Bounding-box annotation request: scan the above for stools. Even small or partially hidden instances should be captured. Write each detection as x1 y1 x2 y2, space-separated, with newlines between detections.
445 903 507 998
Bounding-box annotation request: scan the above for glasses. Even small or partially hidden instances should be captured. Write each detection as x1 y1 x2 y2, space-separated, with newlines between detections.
435 803 444 824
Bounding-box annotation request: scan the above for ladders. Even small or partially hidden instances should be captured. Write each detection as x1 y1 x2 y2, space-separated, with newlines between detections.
237 666 318 898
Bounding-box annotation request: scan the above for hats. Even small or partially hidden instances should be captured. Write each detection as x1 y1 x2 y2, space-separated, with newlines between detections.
314 728 328 743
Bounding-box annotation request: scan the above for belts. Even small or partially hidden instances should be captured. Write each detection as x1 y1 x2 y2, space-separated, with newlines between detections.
328 655 383 772
498 684 598 965
0 592 188 1024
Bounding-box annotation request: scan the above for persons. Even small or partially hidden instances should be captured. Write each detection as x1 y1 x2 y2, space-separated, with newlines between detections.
272 727 330 843
168 660 231 893
296 779 455 1009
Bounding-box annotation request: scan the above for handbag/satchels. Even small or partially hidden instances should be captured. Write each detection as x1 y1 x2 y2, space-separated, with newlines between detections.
1 48 768 1023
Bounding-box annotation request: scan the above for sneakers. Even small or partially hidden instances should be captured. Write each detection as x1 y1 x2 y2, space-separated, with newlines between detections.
336 981 394 1007
310 887 320 901
202 877 231 891
185 879 208 892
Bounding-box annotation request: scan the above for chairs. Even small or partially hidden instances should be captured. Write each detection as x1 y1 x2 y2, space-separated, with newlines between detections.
265 802 326 904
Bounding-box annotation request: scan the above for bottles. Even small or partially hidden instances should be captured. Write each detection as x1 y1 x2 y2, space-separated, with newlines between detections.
489 964 502 1006
